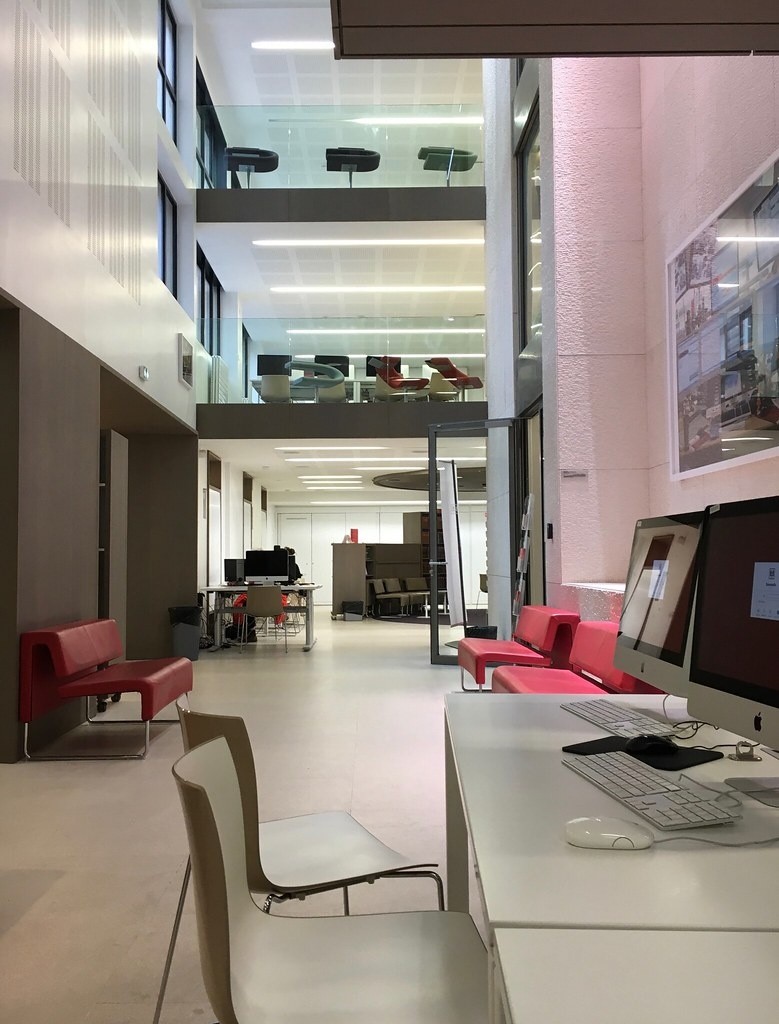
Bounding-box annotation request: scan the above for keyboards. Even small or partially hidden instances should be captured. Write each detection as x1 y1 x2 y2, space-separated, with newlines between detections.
561 751 743 831
560 698 680 739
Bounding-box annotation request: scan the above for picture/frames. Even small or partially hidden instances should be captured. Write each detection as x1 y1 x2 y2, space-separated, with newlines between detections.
664 144 778 483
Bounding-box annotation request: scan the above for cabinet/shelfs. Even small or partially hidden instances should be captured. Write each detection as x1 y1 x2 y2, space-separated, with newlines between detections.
332 543 421 620
403 512 446 590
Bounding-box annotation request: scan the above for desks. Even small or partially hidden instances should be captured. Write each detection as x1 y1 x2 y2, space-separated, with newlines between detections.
446 693 779 934
345 378 377 404
201 581 324 652
489 928 779 1024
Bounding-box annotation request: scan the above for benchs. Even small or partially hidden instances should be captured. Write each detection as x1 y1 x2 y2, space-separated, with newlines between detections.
493 618 666 693
18 615 194 763
458 604 582 692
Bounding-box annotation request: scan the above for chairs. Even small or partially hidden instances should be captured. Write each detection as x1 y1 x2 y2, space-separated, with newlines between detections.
374 372 404 404
476 574 489 607
239 587 289 654
261 374 291 405
318 375 347 404
368 578 430 620
428 372 464 403
153 699 500 1024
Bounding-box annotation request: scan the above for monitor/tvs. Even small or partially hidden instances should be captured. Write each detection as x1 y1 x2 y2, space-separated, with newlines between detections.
612 510 705 698
686 495 779 752
753 181 779 272
257 355 292 376
314 355 349 377
246 548 289 585
366 356 402 377
224 559 247 585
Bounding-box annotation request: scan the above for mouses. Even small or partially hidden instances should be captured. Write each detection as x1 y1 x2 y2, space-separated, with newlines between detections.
565 816 654 850
624 734 679 755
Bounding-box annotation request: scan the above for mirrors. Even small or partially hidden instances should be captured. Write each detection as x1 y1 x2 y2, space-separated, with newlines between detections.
179 334 196 392
